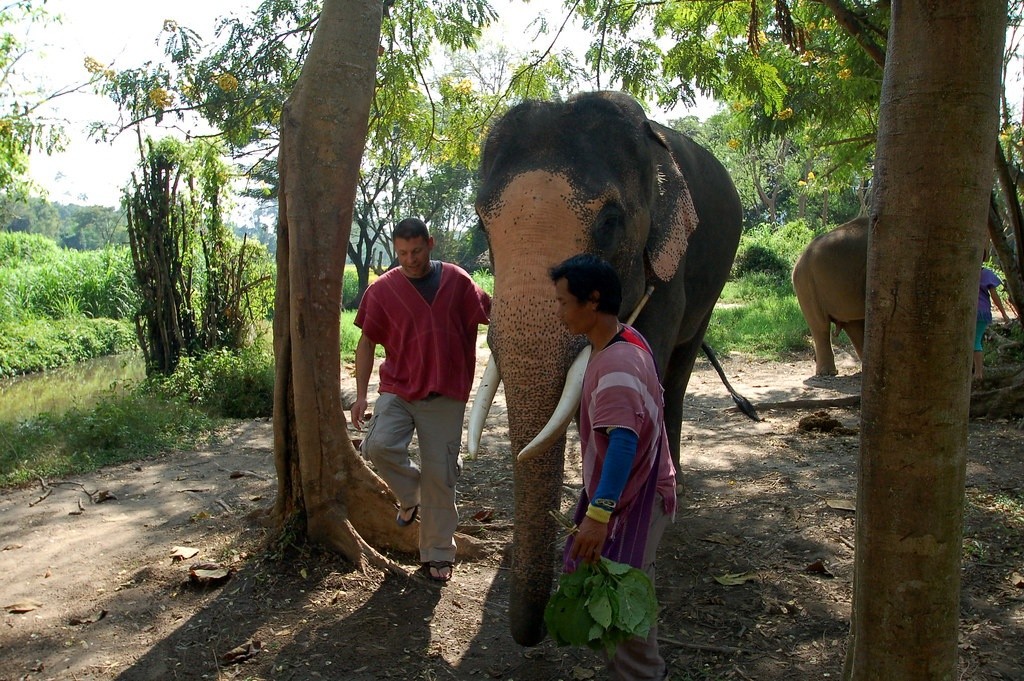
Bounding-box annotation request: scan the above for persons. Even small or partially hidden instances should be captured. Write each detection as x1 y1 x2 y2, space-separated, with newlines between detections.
549 252 678 681
972 249 1010 380
352 216 493 582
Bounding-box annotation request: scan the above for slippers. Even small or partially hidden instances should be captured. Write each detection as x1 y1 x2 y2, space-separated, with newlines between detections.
396 503 418 527
424 559 454 582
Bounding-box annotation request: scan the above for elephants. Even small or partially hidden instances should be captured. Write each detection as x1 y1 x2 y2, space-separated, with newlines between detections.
792 217 870 377
467 90 761 648
